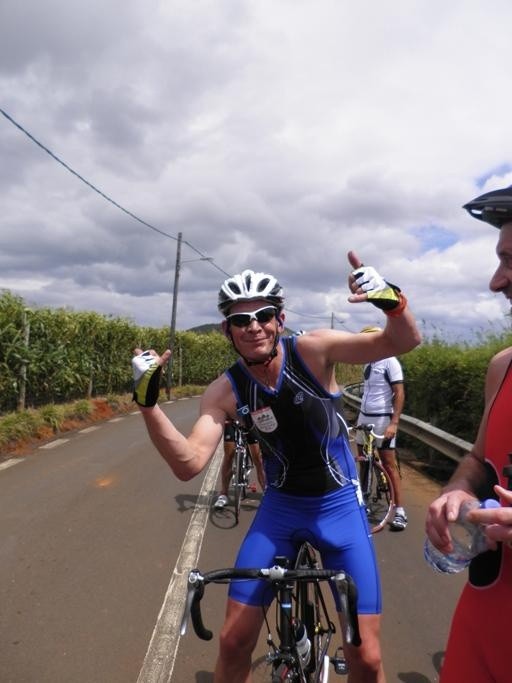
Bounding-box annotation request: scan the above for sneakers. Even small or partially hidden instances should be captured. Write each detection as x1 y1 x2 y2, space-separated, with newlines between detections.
390 512 408 530
214 495 229 508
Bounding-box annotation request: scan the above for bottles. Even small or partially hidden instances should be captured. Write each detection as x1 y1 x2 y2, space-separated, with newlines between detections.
425 499 501 577
374 457 383 465
292 618 311 665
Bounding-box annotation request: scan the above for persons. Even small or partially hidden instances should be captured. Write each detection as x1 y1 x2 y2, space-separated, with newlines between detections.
355 327 408 530
215 416 267 508
130 251 422 681
426 183 511 681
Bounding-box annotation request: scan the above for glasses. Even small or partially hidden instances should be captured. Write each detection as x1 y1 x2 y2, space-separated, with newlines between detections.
226 305 278 329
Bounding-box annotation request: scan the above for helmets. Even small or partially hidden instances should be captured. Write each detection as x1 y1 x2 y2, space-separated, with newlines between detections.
361 326 382 333
462 184 511 228
218 270 285 314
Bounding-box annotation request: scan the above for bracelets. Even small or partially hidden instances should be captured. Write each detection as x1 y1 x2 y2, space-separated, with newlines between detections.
385 293 408 316
